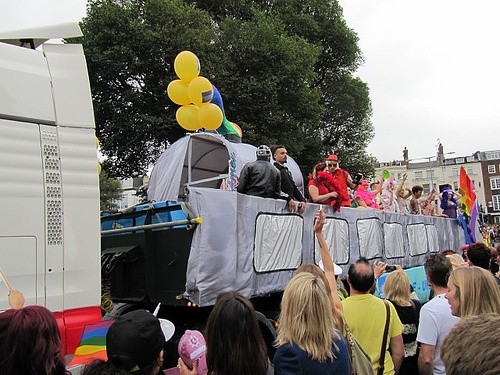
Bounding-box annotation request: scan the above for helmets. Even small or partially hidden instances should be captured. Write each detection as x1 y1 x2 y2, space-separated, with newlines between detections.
256 144 271 157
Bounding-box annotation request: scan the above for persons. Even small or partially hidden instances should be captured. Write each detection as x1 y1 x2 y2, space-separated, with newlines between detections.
81 310 165 375
237 145 281 200
0 289 72 375
308 155 462 219
403 147 408 165
272 145 306 214
439 144 443 155
177 211 500 375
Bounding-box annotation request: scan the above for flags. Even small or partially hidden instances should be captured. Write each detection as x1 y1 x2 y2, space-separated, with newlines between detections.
457 167 478 245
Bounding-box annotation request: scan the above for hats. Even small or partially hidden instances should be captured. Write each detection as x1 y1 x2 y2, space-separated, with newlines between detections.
105 309 175 366
326 155 341 163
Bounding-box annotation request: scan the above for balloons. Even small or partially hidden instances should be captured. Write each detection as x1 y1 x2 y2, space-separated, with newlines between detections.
167 51 224 131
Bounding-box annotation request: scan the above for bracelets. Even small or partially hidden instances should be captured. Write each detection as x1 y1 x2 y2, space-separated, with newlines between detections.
374 278 376 281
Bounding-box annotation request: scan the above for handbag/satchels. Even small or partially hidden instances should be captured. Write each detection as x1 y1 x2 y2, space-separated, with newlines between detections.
340 312 375 375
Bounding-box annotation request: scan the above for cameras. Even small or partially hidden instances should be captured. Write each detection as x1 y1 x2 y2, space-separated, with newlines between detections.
386 266 396 272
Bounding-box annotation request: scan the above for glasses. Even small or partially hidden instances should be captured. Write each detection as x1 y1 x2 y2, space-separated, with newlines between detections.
328 163 337 167
